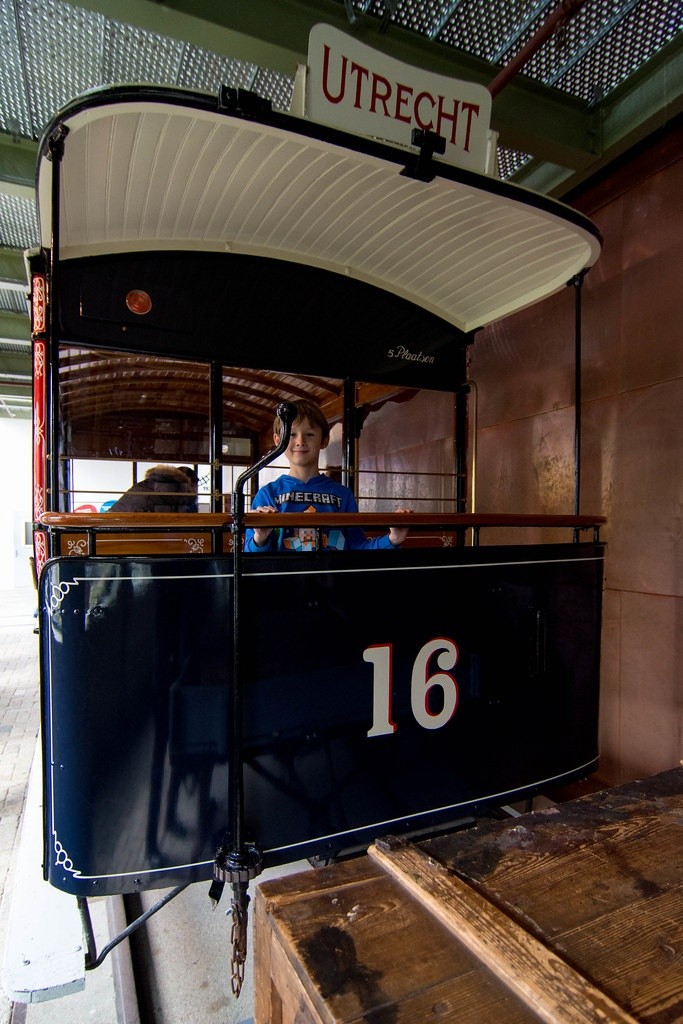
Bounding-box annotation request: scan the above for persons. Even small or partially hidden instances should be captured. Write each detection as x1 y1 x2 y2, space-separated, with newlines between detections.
104 464 198 513
239 398 418 557
